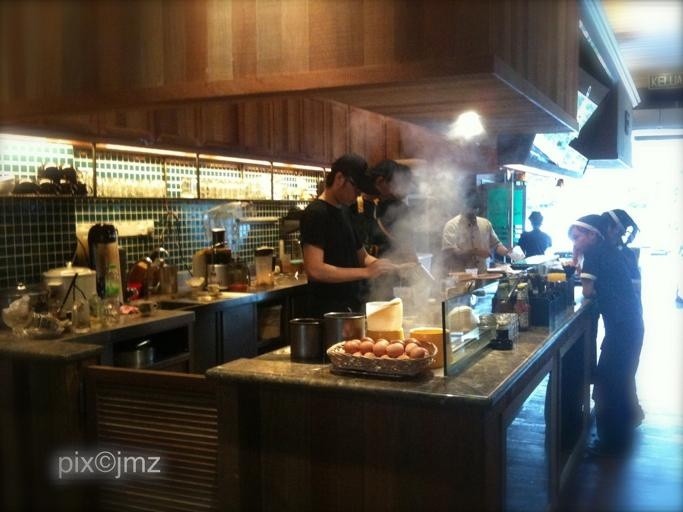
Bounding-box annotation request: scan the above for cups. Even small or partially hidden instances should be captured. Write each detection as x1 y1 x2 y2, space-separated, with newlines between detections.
409 328 454 370
179 172 285 200
87 175 167 199
69 296 91 335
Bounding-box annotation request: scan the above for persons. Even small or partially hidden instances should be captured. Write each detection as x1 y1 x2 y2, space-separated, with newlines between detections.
599 209 643 315
295 152 422 315
567 215 645 442
359 160 556 302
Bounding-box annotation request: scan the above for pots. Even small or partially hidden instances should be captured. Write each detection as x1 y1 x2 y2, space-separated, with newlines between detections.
112 339 155 365
323 310 365 349
38 260 97 309
289 317 326 361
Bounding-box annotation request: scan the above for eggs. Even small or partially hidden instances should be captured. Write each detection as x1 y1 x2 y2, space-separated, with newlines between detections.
344 336 425 359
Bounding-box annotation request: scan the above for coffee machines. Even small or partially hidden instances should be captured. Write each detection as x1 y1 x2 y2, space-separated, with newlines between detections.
204 227 250 291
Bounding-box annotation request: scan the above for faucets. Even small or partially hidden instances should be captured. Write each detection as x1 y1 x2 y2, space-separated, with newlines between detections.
144 248 170 289
124 255 151 297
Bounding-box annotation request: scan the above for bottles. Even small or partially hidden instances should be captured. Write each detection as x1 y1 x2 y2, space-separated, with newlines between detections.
513 283 530 329
102 261 120 303
494 312 519 340
254 246 273 290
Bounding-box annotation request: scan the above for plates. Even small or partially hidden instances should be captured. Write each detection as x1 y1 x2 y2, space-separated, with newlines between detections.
23 327 64 340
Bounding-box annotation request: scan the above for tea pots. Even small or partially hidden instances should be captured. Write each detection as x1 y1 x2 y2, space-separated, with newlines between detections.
0 161 92 198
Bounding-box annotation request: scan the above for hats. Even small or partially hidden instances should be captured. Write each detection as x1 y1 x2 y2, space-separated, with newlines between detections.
573 215 607 240
333 154 381 196
609 209 637 244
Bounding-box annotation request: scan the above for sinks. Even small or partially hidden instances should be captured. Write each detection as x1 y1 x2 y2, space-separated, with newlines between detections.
157 300 195 311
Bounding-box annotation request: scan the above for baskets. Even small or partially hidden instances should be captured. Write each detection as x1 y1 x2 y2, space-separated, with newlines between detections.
326 338 439 377
